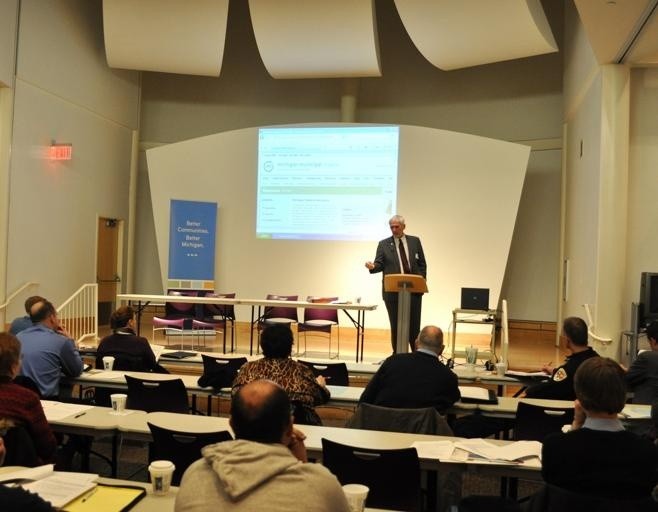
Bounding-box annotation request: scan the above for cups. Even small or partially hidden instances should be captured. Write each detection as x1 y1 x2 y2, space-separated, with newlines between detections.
341 483 370 512
495 363 507 378
148 459 176 496
110 393 128 413
464 346 479 365
102 356 115 372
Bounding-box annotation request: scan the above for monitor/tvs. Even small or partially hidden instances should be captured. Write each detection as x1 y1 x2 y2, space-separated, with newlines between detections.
639 272 658 328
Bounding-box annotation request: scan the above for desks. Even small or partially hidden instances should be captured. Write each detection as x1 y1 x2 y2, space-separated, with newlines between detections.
77 341 556 388
2 463 395 510
40 398 546 473
79 365 579 426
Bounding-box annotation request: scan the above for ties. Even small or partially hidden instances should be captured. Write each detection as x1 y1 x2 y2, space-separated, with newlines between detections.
398 238 411 274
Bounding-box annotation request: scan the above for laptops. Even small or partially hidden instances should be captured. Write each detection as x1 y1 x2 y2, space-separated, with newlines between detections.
461 288 489 311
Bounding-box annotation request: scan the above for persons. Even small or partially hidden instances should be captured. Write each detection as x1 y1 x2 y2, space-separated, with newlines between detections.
9 295 83 396
366 214 426 354
95 306 166 408
231 324 330 426
1 332 89 466
523 317 658 511
1 419 61 511
357 326 460 416
172 379 350 511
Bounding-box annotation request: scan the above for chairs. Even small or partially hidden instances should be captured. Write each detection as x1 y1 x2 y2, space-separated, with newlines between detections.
295 359 351 389
513 399 576 442
199 354 248 387
235 391 323 426
357 400 436 433
143 419 232 480
124 375 192 414
15 373 43 399
534 464 657 511
0 420 57 463
116 287 377 361
316 435 425 510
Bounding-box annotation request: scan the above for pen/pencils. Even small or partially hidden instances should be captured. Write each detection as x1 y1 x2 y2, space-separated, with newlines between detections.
517 460 525 463
75 413 86 418
82 488 98 502
548 359 554 366
